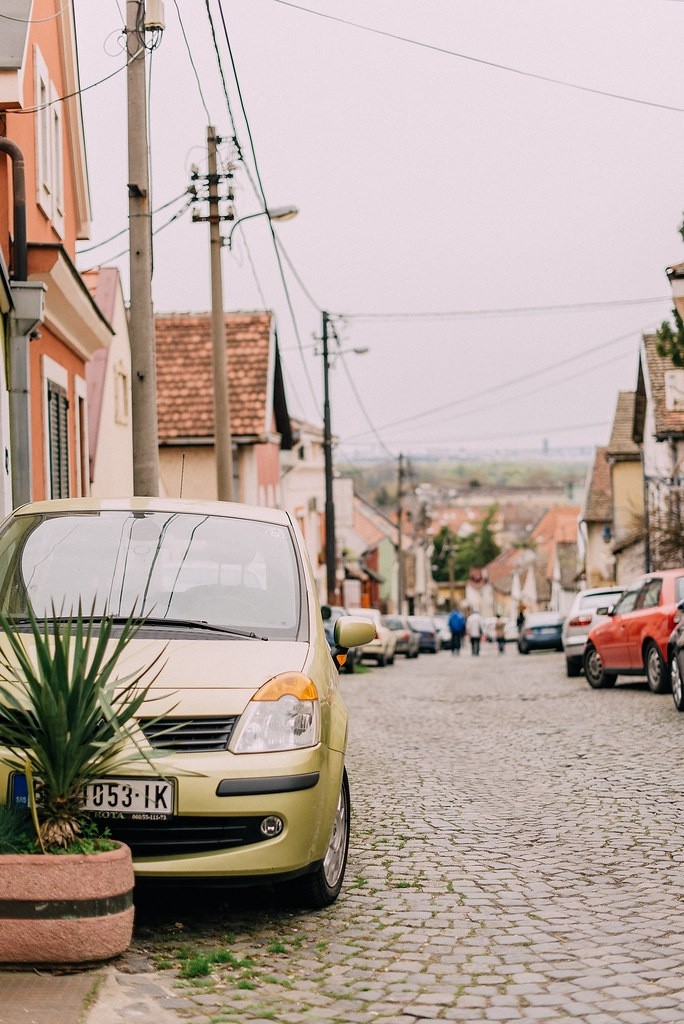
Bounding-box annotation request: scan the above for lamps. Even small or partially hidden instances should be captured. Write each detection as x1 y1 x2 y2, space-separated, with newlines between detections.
603 527 612 544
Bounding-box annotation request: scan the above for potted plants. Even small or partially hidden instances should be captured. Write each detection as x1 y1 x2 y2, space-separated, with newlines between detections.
0 592 206 966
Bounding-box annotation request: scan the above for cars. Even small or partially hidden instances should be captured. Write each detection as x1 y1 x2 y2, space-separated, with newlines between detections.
667 601 684 712
517 611 565 654
1 494 377 914
321 604 453 674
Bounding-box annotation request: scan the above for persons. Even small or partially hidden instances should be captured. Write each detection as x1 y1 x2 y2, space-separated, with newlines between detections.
448 606 466 658
493 614 507 653
517 612 525 634
466 610 486 656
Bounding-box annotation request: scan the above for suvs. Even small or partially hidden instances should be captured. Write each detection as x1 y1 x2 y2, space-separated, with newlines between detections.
561 586 628 678
582 567 684 694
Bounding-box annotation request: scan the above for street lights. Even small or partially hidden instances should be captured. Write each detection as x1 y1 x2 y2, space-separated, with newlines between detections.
210 204 301 502
325 345 370 606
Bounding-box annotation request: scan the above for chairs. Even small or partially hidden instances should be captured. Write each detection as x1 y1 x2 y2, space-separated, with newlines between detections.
181 538 275 618
